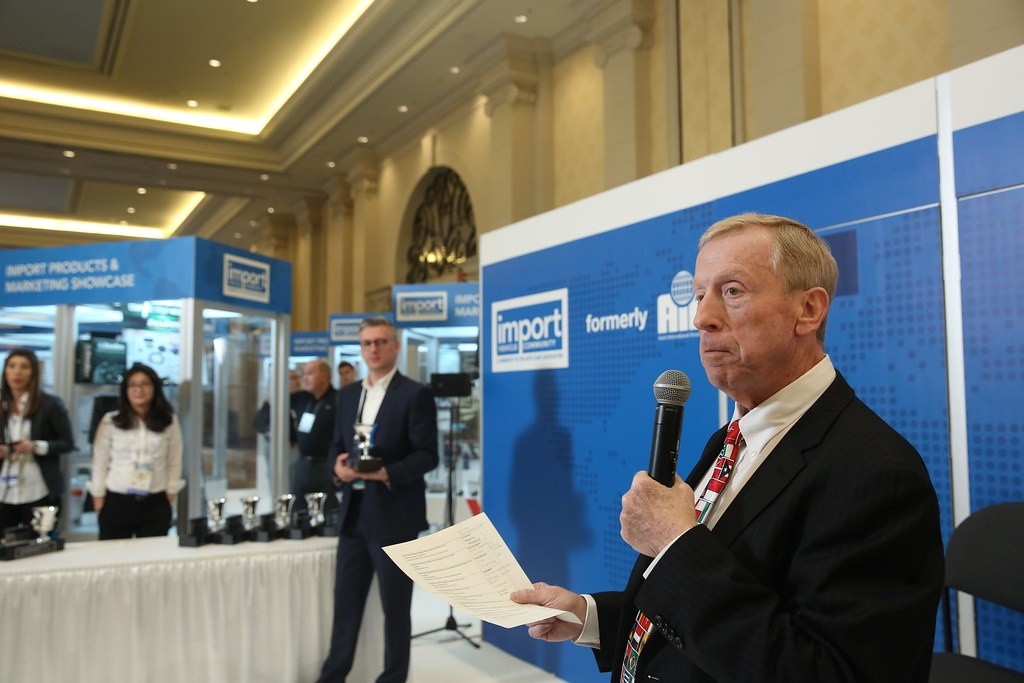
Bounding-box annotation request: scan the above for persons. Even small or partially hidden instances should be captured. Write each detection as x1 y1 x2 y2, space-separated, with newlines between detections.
510 213 946 683
0 348 76 539
86 364 187 540
254 361 358 511
315 316 440 683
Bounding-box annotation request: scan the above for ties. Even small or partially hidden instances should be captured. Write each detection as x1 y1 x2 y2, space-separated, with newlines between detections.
619 420 745 683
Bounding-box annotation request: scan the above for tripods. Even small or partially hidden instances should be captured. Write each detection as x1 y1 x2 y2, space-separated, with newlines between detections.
412 397 481 650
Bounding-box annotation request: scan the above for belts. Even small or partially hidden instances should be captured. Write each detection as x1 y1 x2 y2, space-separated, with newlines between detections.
304 455 326 461
350 480 383 490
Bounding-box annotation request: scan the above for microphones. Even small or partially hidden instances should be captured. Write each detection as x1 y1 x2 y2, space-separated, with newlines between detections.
649 368 689 487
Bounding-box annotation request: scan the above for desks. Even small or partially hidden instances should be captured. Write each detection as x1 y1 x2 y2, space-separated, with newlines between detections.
0 537 384 683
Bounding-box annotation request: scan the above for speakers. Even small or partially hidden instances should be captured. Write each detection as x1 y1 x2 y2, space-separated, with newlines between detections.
431 372 474 396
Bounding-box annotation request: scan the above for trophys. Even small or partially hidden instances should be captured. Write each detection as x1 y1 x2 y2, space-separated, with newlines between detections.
345 424 383 474
31 506 58 545
206 492 328 534
